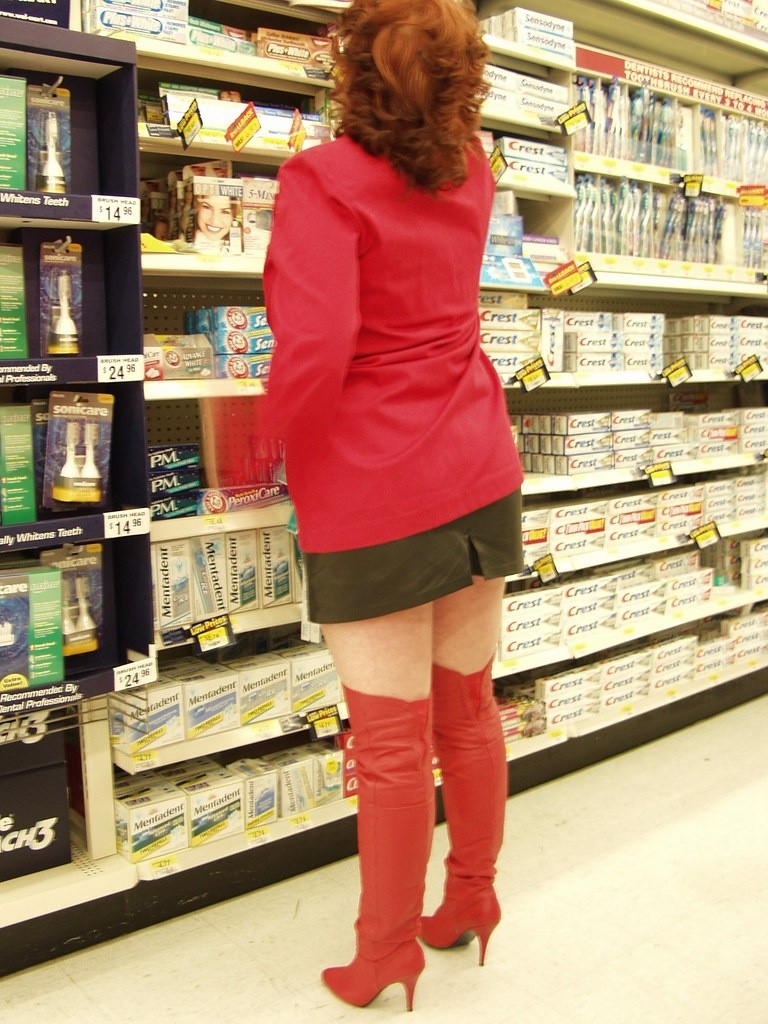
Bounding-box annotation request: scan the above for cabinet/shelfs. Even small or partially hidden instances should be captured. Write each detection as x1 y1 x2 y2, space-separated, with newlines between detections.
0 0 767 932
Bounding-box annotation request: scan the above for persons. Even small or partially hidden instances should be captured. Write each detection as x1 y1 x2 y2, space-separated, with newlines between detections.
257 0 525 1012
218 91 241 103
141 185 237 252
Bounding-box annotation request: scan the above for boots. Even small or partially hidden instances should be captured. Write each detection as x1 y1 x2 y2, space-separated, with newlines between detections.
322 682 425 1012
418 660 507 966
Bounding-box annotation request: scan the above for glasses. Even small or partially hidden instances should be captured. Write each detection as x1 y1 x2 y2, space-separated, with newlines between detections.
328 28 357 60
